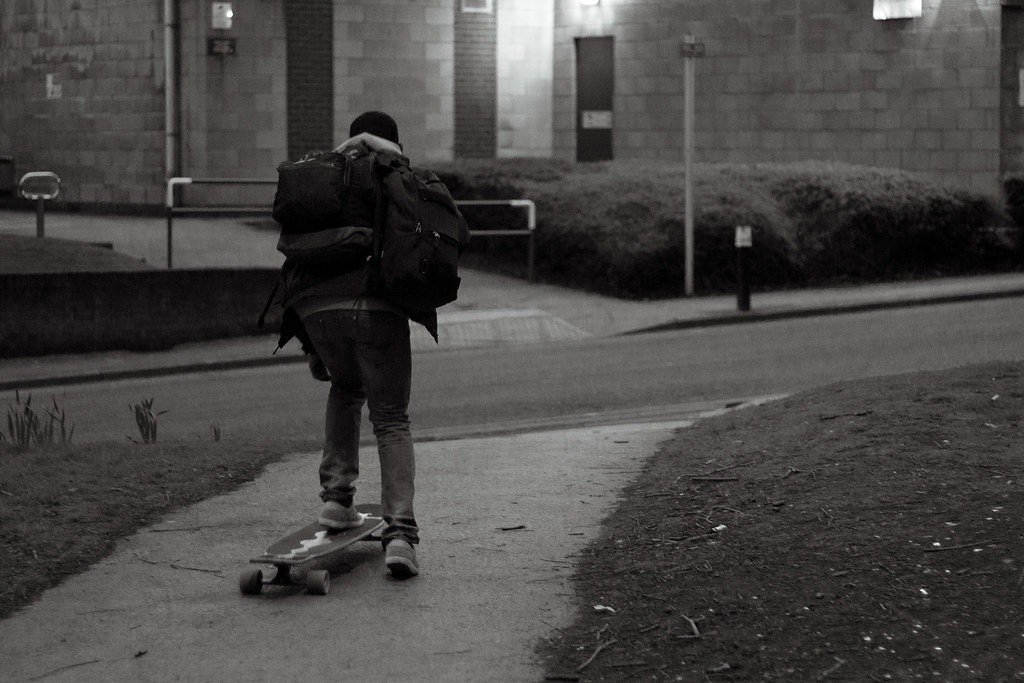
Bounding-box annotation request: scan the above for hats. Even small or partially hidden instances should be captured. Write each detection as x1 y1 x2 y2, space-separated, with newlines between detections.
349 111 398 145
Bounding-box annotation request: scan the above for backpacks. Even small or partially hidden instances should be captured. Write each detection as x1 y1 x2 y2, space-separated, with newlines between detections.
368 167 471 310
274 151 374 260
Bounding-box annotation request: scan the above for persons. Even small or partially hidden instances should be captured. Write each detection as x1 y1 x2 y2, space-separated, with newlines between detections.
271 111 472 580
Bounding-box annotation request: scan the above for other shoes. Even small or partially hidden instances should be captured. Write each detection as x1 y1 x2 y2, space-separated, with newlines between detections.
319 500 364 528
385 540 419 577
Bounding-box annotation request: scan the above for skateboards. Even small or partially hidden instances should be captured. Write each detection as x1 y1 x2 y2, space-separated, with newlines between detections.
236 503 388 595
